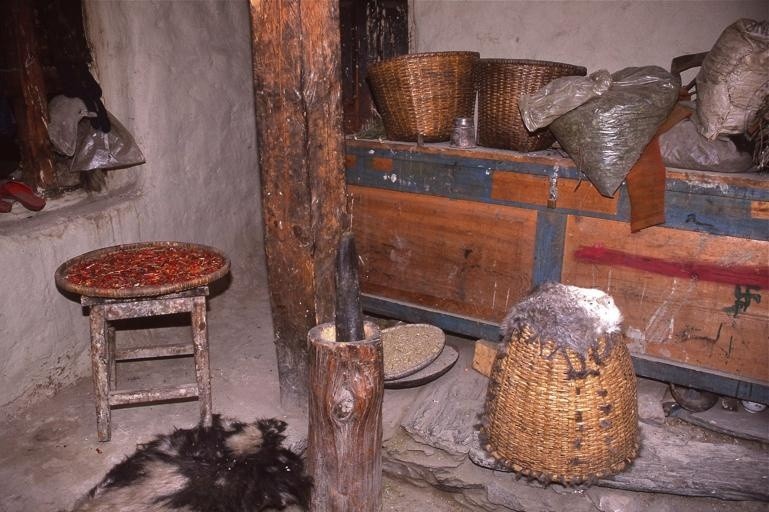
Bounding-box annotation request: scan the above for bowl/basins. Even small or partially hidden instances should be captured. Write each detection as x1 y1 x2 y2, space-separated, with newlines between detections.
741 400 767 414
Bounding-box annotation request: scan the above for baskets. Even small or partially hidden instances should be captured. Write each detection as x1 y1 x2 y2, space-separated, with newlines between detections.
479 325 643 485
476 57 588 155
366 51 481 144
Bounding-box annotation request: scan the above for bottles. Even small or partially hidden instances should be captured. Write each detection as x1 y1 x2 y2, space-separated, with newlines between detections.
449 115 477 151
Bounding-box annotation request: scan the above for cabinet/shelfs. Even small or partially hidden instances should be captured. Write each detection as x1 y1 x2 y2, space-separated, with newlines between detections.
344 137 767 405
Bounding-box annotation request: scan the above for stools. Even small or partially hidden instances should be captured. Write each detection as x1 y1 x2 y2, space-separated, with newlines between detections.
80 285 211 440
